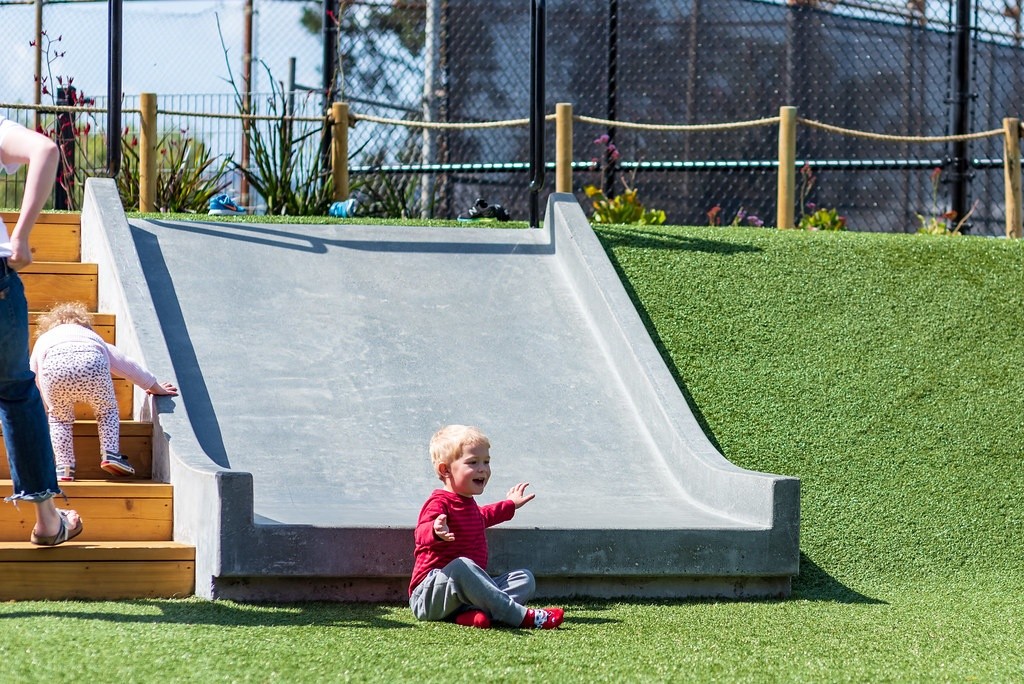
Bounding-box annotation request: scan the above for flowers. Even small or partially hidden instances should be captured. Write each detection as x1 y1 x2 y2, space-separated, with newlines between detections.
586 133 667 224
917 168 979 236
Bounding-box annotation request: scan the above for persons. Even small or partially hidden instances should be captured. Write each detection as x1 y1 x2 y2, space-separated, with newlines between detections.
407 424 566 629
0 114 83 548
28 299 180 481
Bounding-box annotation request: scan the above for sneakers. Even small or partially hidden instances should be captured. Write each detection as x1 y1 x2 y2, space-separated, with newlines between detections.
457 198 509 221
56 464 76 481
209 194 246 216
330 199 358 217
100 450 135 476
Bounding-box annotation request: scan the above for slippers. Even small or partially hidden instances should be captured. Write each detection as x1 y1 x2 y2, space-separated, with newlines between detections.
30 508 83 546
